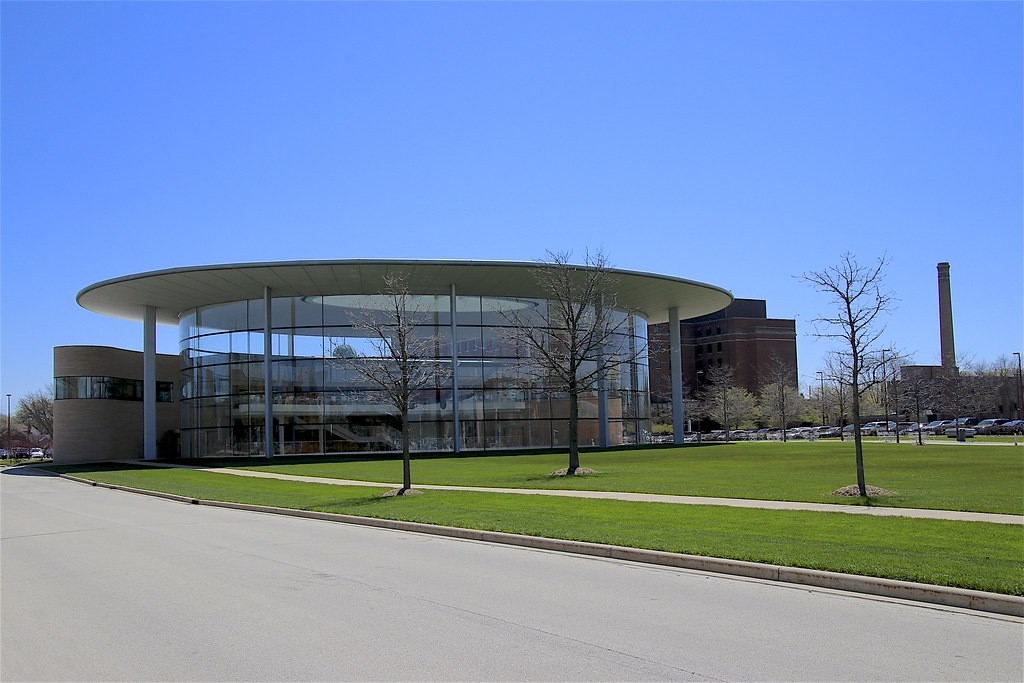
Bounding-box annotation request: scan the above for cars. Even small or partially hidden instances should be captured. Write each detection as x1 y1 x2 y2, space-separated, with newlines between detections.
973 419 1010 435
877 422 917 434
647 419 897 440
899 423 928 436
997 420 1024 435
419 436 491 450
0 448 53 460
921 420 953 435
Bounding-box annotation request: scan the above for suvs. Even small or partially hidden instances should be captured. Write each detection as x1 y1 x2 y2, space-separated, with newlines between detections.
941 417 982 432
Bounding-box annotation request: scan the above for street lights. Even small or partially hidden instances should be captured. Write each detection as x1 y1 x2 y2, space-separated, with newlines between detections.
816 371 825 426
883 349 890 432
696 370 703 391
1012 352 1023 407
6 394 12 435
